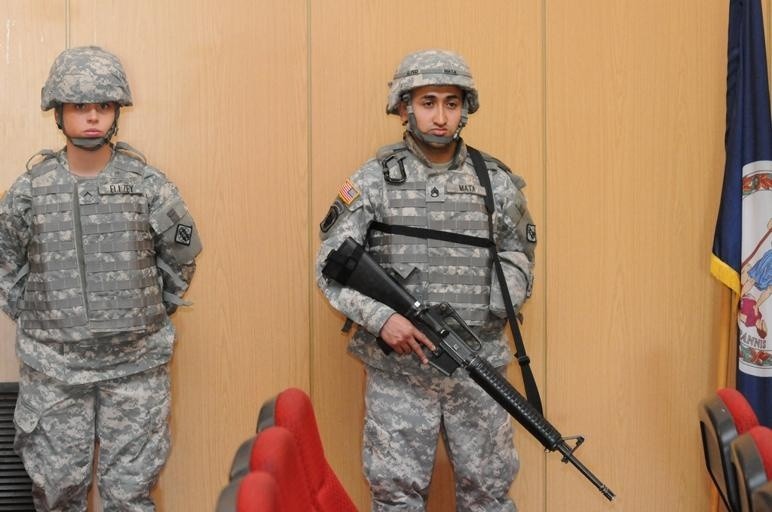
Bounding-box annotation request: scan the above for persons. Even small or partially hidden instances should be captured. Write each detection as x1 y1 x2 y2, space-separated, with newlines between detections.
1 46 202 512
315 49 536 511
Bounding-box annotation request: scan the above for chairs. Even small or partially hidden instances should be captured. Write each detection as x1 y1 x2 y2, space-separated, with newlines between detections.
730 425 772 511
216 470 280 512
698 388 761 511
229 426 317 512
256 388 364 511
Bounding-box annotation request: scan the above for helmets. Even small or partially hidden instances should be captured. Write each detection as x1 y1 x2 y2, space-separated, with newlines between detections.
386 49 478 118
38 44 134 110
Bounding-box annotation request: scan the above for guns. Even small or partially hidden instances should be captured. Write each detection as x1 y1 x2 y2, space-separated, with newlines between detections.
322 236 618 500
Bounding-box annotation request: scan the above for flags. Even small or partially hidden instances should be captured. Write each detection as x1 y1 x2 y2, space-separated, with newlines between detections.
709 0 772 427
